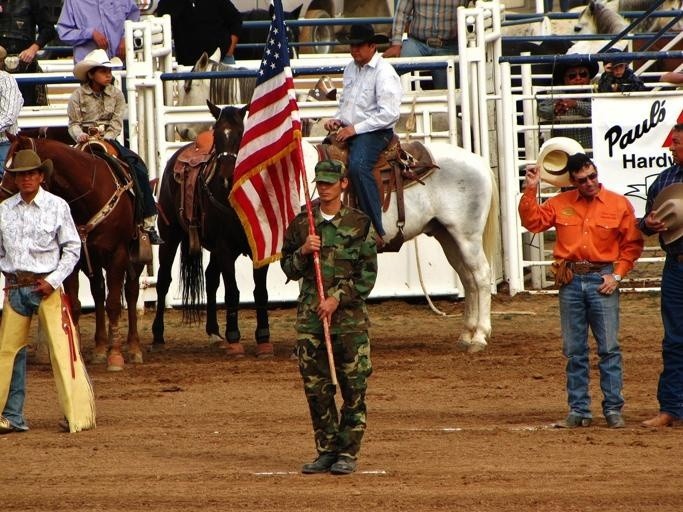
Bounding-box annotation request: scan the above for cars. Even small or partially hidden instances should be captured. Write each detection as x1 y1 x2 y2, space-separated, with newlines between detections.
134 0 348 57
34 1 72 59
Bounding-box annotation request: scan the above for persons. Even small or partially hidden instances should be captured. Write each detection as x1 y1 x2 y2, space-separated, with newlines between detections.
278 158 378 474
1 149 83 432
516 154 646 431
534 47 681 190
322 21 403 251
0 1 241 244
637 123 682 429
383 1 476 91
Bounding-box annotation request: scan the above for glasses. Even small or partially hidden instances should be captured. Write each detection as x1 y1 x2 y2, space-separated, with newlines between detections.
578 173 596 183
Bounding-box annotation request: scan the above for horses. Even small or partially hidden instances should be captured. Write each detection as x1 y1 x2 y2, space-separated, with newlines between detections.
0 127 147 375
150 95 277 359
297 135 498 357
176 45 333 142
565 1 632 75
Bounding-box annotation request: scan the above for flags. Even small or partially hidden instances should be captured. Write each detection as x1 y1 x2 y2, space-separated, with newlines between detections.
228 0 304 266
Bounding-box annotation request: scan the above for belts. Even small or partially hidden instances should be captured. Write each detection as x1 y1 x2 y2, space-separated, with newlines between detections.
414 37 455 47
574 263 611 272
5 271 48 285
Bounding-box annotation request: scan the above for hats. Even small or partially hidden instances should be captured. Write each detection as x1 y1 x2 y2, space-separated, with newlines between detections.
335 24 389 44
554 49 626 85
652 182 683 245
73 49 123 80
4 149 53 176
312 159 346 184
537 137 585 186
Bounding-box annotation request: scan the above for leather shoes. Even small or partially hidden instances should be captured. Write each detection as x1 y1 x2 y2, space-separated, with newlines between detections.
606 415 625 428
641 412 672 427
303 454 357 474
555 415 592 428
149 227 165 244
0 417 24 433
